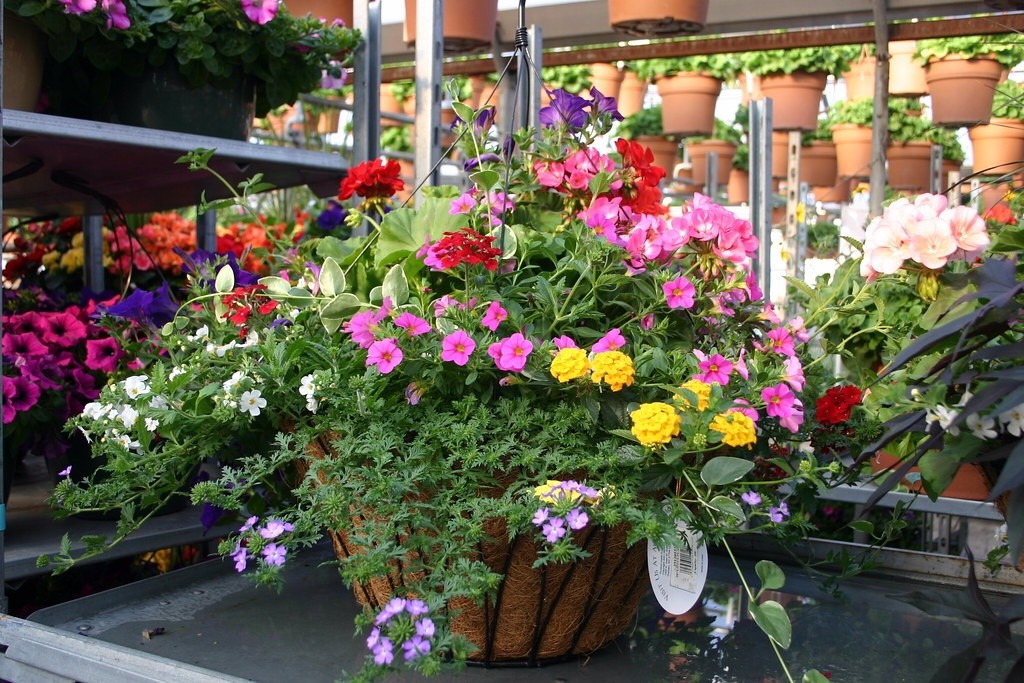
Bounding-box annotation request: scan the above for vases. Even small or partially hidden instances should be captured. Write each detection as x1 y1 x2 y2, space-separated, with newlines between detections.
136 63 258 142
1 23 47 112
280 1 354 33
283 422 656 667
404 0 497 46
607 0 709 38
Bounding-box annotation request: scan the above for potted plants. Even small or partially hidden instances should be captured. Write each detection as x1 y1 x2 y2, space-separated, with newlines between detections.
264 25 1024 206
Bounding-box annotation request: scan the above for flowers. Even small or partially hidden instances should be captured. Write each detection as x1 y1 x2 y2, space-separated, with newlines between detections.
1 206 314 445
851 159 1024 576
1 0 365 119
35 83 890 682
